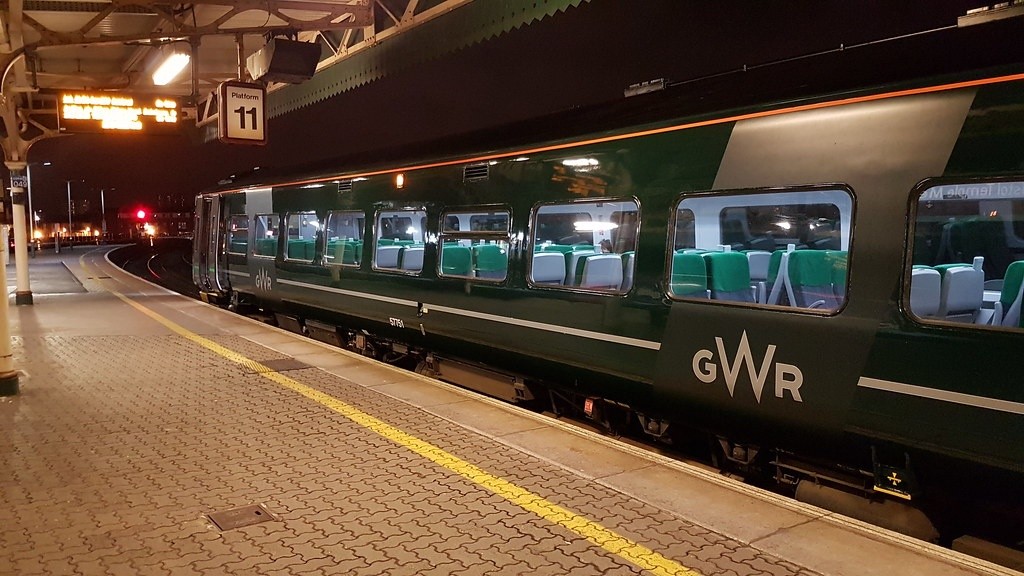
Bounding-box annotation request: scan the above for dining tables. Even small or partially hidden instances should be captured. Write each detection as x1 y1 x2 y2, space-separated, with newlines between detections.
327 244 357 265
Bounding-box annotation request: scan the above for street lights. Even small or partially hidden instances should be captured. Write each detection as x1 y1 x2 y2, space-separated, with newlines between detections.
67 178 85 251
101 187 117 245
27 162 51 256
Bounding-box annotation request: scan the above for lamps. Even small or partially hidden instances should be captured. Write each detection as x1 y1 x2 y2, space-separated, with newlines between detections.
246 27 323 85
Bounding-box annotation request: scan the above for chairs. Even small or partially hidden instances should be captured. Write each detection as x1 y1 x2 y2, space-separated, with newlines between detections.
442 246 476 277
759 249 787 305
744 250 773 303
830 250 848 305
620 251 635 291
672 254 711 299
910 265 941 319
783 250 838 309
377 245 403 268
573 253 623 290
986 260 1024 328
533 251 566 286
703 251 756 303
231 235 315 260
472 245 508 278
934 263 985 324
396 247 424 271
355 244 363 265
564 249 603 286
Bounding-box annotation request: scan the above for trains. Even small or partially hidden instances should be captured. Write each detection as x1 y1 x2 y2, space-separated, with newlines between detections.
191 1 1024 536
